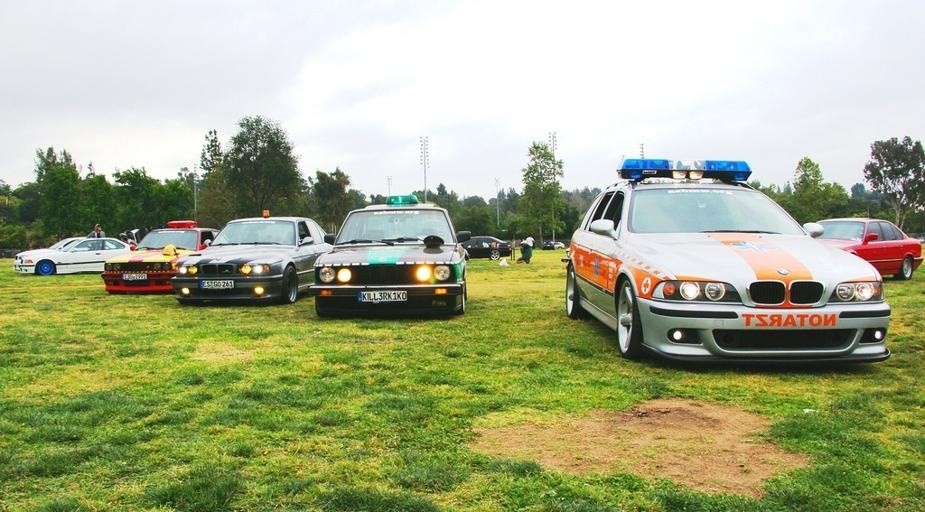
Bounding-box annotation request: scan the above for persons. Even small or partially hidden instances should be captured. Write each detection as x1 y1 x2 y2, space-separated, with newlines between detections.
86 223 106 250
120 236 137 248
517 235 536 264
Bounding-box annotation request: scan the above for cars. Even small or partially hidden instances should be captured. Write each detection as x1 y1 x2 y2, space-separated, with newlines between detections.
543 240 565 250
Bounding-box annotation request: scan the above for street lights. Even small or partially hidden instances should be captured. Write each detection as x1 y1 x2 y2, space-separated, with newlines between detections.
419 135 430 204
548 131 557 239
493 177 501 226
386 176 393 195
191 163 200 221
